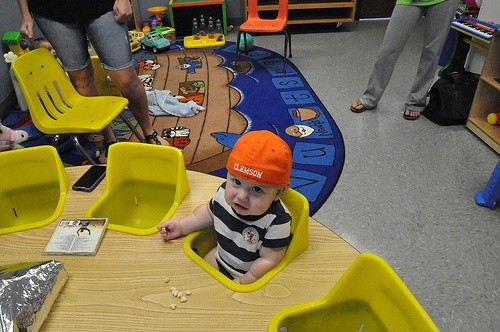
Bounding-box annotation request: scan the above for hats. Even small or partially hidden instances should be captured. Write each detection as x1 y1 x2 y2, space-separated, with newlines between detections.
226 130 292 186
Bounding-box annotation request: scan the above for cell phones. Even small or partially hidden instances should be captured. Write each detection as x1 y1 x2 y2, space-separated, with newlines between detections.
72 166 108 192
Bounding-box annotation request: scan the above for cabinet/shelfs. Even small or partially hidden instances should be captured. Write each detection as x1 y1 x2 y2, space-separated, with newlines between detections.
466 28 500 155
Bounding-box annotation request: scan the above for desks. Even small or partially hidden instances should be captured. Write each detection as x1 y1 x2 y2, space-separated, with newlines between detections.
0 162 440 332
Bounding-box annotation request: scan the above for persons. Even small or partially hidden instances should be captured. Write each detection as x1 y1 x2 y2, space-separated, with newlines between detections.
158 130 293 284
350 0 462 120
17 0 167 161
29 37 66 71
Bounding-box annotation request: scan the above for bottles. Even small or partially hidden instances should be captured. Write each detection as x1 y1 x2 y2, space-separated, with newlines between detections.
192 15 223 34
142 23 150 32
487 112 500 125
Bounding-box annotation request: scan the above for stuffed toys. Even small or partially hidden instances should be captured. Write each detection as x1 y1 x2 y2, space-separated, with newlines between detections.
0 125 29 153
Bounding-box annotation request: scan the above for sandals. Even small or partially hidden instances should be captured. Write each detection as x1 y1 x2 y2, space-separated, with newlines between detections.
102 139 119 150
350 99 364 113
403 110 420 120
145 129 169 146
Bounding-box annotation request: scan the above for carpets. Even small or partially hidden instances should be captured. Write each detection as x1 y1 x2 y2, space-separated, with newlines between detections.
0 38 345 218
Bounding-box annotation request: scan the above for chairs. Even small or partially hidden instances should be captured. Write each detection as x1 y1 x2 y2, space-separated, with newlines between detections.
234 0 292 74
11 47 144 166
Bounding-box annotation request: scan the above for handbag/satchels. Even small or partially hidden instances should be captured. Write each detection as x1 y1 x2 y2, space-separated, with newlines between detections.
420 70 481 126
